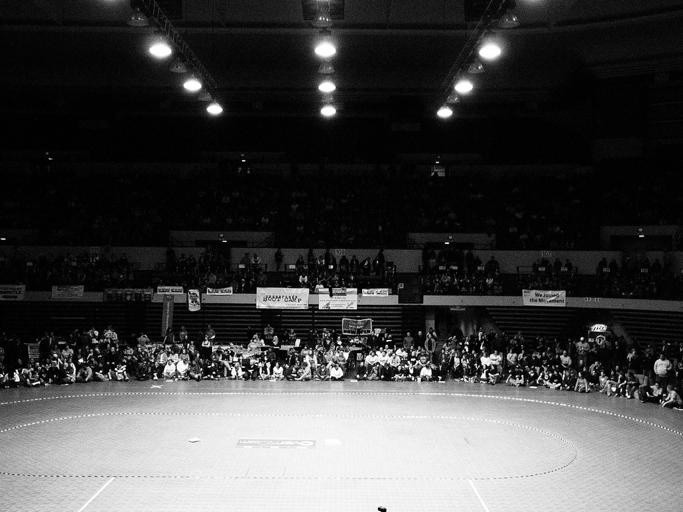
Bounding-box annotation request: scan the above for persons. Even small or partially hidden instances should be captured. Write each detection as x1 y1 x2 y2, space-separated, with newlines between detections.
0 159 682 410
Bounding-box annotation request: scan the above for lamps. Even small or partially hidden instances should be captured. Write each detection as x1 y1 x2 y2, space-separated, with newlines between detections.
126 11 148 26
468 61 485 75
496 11 519 28
316 60 335 73
310 5 334 27
169 58 186 72
197 89 213 102
